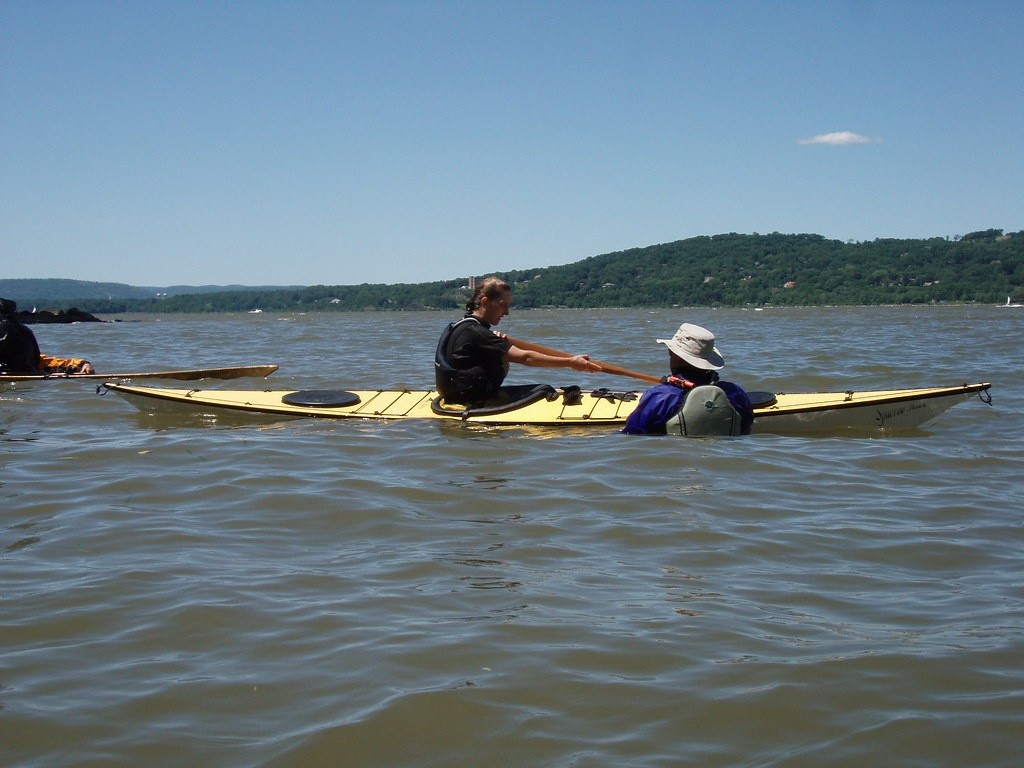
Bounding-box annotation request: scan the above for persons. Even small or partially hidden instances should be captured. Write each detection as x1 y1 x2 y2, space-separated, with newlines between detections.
434 277 603 405
0 319 95 374
616 323 754 437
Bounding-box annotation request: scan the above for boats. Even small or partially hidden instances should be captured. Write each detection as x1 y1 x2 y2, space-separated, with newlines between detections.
97 382 996 433
1 365 279 392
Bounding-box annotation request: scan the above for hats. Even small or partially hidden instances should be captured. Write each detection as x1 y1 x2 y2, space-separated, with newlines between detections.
655 322 726 371
